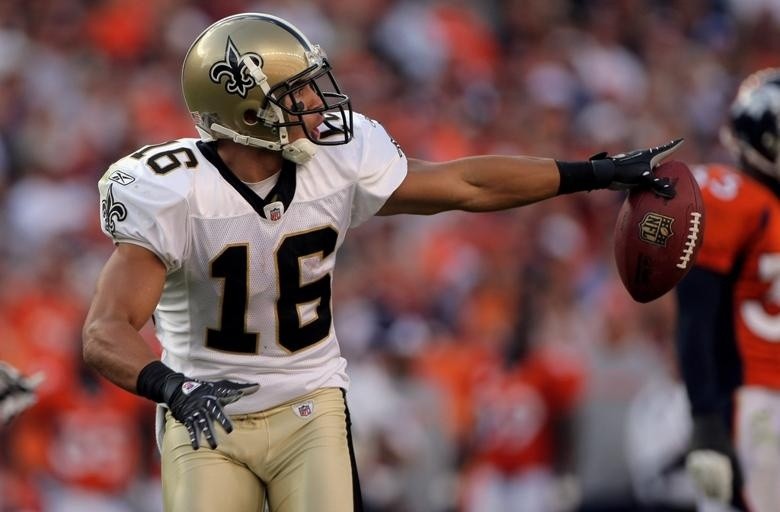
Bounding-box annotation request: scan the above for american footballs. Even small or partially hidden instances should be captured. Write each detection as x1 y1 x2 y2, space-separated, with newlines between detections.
614 160 704 303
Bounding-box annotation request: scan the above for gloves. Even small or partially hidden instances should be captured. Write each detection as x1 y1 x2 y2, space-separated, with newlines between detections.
160 370 261 454
587 135 689 203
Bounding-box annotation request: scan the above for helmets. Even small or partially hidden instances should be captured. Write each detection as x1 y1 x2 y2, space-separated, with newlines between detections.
179 11 329 147
717 67 779 178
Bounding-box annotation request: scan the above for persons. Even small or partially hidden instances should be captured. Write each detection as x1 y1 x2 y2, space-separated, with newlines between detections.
1 1 778 512
